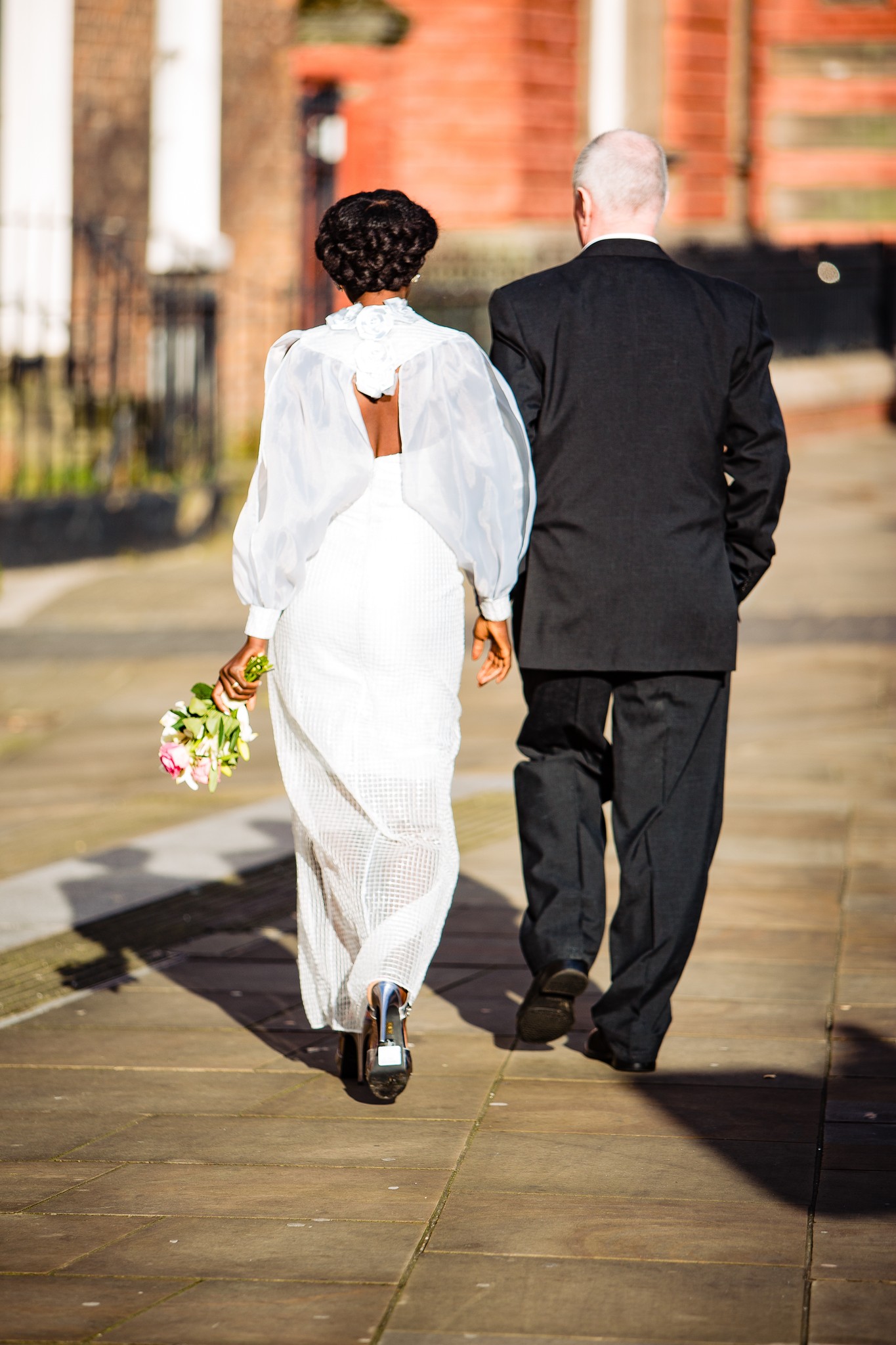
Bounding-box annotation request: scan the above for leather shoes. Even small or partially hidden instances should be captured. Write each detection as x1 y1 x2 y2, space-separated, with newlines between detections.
514 960 590 1042
582 1024 656 1071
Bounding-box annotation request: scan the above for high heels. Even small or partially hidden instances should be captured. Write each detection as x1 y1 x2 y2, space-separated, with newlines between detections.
366 983 412 1102
332 1021 365 1082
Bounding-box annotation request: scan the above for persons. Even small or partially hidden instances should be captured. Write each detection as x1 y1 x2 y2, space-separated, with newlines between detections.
211 188 535 1103
489 129 790 1072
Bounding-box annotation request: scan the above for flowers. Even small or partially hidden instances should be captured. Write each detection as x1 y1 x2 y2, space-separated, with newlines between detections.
156 656 276 794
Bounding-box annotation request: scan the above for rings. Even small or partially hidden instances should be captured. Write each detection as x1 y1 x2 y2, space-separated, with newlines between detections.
232 682 239 689
